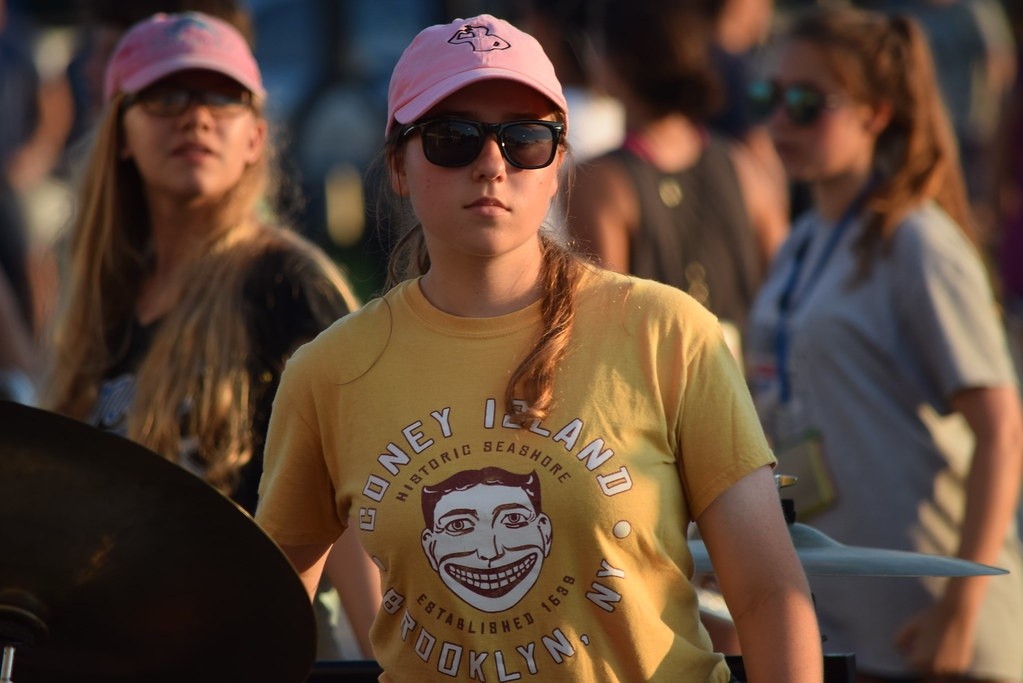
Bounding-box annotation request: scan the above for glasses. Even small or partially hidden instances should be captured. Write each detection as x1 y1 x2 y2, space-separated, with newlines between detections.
746 80 827 123
139 85 258 117
397 115 565 169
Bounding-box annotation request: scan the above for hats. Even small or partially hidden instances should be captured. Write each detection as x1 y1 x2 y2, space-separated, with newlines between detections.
104 11 264 111
384 14 568 144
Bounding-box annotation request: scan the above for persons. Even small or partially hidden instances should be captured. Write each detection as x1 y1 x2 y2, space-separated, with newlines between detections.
0 0 1023 683
251 12 826 682
